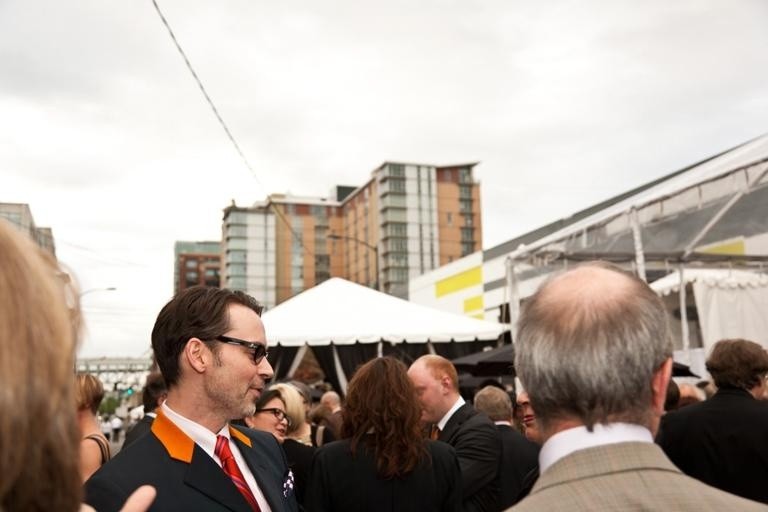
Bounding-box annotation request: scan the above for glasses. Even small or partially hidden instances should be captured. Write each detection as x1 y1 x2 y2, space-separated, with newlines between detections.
216 334 269 364
256 407 291 424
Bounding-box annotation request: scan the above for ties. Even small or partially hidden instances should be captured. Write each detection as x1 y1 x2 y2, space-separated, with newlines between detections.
430 425 440 442
214 434 262 512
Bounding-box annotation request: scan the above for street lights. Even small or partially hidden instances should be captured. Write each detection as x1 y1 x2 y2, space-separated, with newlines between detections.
326 234 380 291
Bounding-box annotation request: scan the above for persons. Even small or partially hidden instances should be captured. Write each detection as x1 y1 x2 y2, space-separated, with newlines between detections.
1 212 768 512
